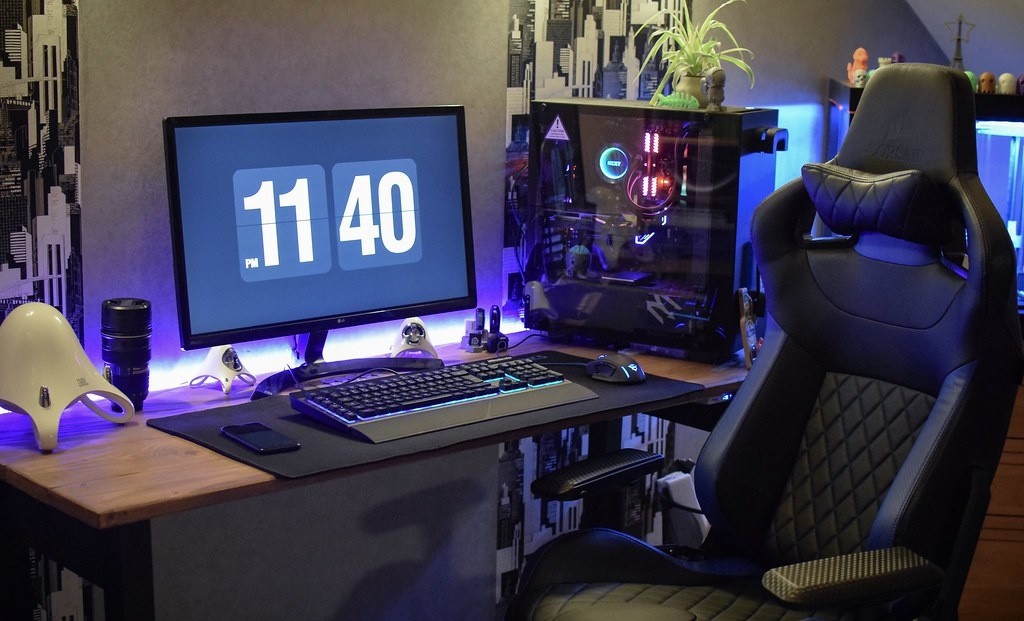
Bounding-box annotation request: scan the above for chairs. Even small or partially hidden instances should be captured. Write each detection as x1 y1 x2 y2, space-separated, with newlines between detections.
506 62 1024 621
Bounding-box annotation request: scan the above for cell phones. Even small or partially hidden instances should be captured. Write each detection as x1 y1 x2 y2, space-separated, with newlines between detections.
222 421 302 454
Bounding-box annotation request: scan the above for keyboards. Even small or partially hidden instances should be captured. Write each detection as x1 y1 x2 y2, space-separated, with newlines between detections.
289 356 599 443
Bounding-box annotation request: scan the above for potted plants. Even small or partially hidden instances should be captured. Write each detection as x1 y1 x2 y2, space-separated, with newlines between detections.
630 0 756 108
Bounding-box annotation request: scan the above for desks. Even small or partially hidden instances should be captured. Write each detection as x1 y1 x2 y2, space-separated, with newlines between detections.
0 329 755 621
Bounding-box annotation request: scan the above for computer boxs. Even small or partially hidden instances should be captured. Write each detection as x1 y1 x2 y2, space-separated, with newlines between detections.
525 98 789 364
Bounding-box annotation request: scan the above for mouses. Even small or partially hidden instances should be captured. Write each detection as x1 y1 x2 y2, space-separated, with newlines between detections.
584 354 646 384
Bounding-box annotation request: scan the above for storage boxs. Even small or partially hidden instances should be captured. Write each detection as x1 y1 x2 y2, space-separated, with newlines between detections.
824 77 1024 321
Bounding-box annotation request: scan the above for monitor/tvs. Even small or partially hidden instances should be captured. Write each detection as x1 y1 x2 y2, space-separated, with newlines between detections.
164 105 477 402
